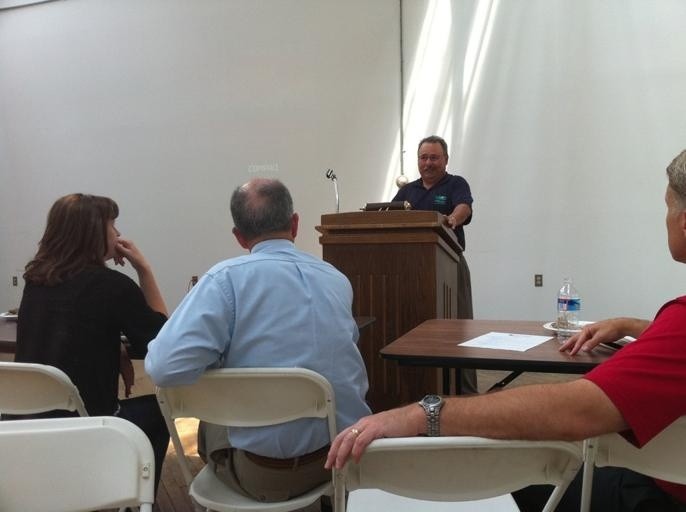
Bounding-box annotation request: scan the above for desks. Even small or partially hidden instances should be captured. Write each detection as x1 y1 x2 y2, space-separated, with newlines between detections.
0 312 376 363
381 316 641 396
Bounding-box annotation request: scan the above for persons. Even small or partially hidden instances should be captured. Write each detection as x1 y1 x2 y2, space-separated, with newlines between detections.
323 149 686 511
13 192 169 511
384 134 475 257
144 177 378 503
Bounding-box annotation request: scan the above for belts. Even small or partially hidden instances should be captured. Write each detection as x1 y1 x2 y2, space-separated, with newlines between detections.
244 443 330 468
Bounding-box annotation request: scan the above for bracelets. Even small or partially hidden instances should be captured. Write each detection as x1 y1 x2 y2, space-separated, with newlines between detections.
451 213 459 224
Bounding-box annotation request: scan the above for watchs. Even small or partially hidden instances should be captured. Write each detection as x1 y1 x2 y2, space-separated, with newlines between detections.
417 387 446 439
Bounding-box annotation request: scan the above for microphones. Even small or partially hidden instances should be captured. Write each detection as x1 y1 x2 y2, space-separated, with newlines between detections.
326 169 339 213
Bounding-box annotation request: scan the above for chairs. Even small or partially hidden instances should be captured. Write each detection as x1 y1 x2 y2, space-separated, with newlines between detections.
330 434 613 512
0 362 89 416
156 366 338 511
0 415 155 512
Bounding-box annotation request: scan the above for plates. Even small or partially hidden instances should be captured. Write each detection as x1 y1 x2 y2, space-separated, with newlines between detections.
542 320 601 334
0 310 18 321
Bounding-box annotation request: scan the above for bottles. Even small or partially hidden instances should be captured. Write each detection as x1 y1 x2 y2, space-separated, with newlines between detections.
557 276 581 343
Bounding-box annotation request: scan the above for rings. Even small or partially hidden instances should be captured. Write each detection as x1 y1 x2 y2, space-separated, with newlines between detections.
347 426 362 436
